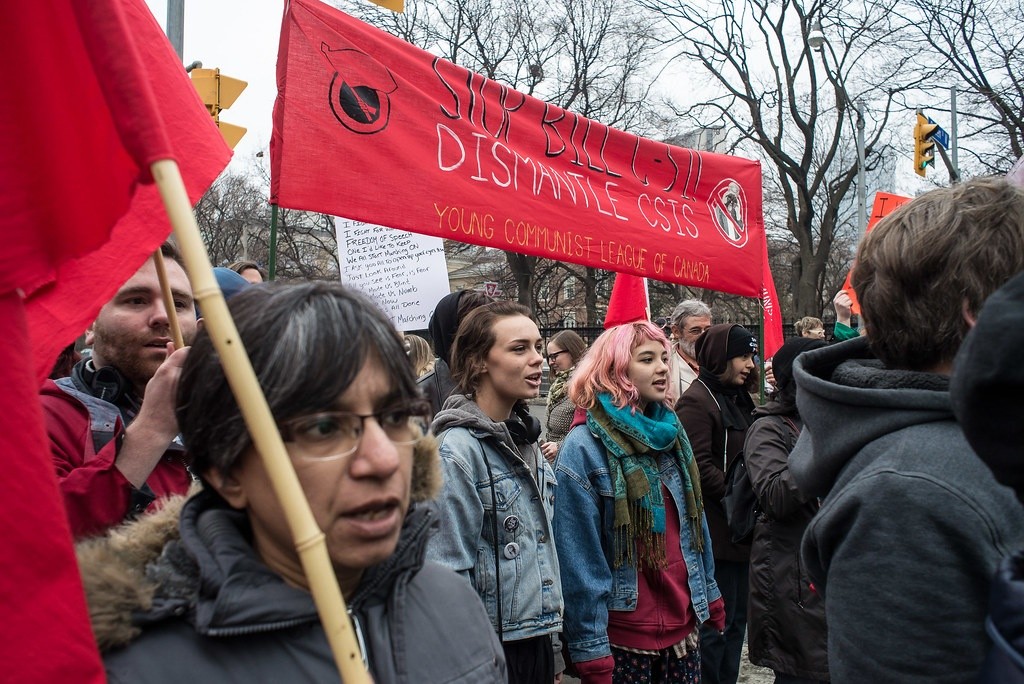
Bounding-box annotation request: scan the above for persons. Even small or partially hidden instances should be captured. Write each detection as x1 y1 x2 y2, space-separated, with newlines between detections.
38 178 1024 684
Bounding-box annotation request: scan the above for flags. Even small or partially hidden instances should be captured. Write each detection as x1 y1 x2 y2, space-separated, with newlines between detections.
605 274 648 327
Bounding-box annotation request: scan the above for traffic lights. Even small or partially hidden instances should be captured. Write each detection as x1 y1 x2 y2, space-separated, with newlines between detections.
914 114 939 178
191 66 248 151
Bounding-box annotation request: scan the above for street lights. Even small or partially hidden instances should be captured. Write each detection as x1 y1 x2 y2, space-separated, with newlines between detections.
806 19 867 242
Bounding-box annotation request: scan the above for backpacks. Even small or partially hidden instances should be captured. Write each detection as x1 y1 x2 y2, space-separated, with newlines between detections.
721 412 795 538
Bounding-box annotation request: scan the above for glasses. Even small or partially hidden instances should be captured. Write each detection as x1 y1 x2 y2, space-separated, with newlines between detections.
807 329 825 337
280 399 434 464
547 348 571 362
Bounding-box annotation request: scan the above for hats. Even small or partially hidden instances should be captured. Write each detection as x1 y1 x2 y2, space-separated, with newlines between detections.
727 326 760 359
772 337 831 391
195 267 250 319
949 271 1023 497
